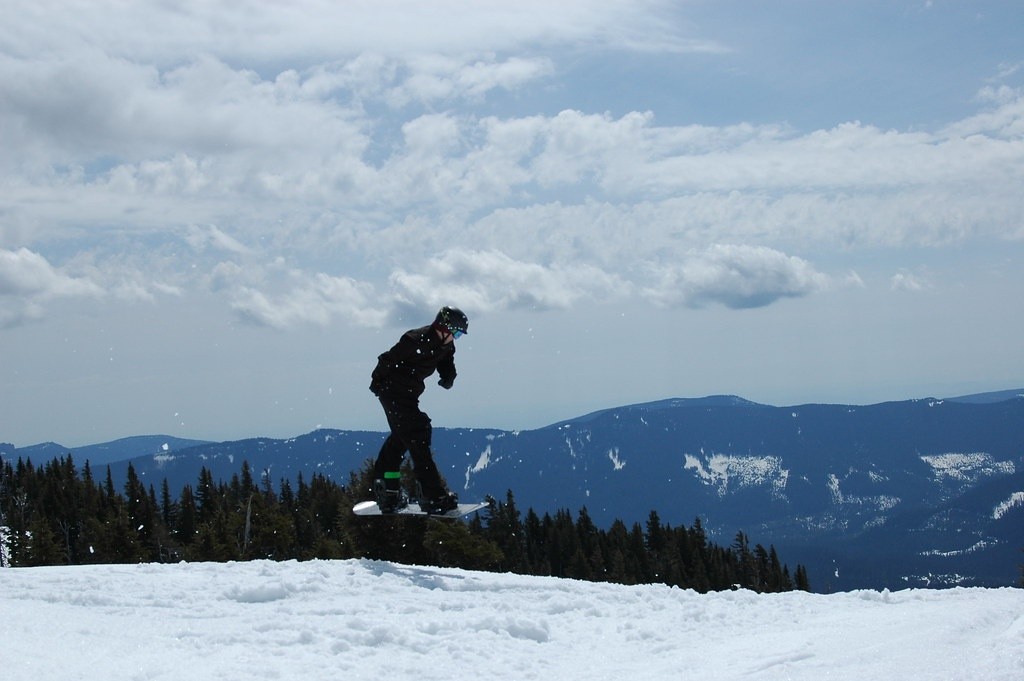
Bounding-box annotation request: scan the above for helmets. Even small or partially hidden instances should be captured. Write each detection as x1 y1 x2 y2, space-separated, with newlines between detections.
436 306 468 335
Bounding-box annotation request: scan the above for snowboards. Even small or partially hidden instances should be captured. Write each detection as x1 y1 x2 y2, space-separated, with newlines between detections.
352 500 490 518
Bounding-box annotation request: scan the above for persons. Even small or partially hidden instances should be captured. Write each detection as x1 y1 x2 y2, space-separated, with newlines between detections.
369 307 469 516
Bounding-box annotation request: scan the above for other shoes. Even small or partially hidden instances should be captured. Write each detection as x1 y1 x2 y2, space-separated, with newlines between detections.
424 494 458 511
375 490 409 511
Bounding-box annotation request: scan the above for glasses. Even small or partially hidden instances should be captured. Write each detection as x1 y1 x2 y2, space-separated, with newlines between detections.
448 329 464 339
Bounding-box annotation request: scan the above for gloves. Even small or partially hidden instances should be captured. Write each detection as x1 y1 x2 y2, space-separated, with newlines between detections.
438 379 453 389
369 379 382 397
